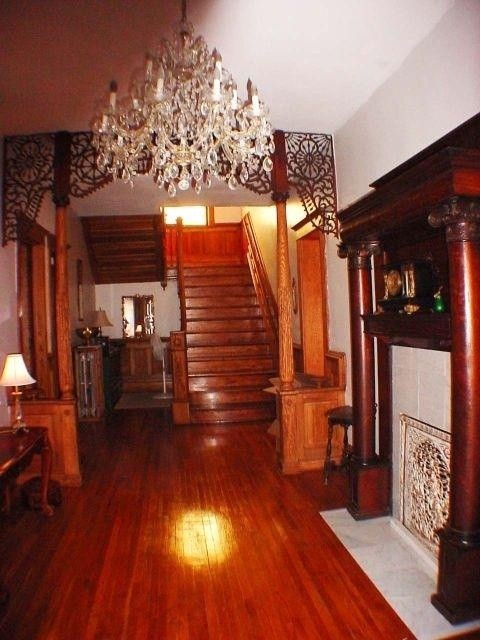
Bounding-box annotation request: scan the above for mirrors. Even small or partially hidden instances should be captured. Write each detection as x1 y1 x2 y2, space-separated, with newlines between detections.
121 294 155 344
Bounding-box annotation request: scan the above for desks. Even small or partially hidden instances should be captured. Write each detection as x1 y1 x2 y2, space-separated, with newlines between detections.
322 406 353 486
1 426 55 518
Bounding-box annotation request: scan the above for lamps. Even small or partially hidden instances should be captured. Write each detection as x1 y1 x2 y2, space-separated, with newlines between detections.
88 0 275 198
0 354 39 434
87 309 111 343
